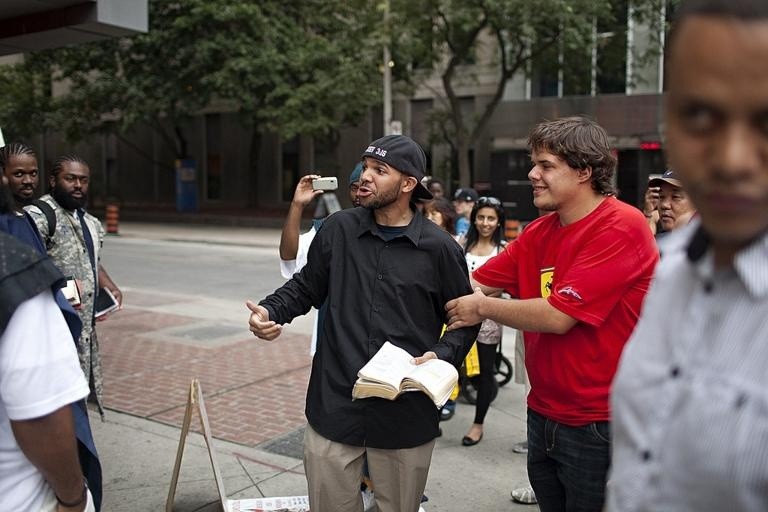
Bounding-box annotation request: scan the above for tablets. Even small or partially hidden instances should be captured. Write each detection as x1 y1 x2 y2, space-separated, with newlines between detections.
93 286 119 318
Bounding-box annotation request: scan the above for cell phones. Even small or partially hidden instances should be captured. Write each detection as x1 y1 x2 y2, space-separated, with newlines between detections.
312 176 338 191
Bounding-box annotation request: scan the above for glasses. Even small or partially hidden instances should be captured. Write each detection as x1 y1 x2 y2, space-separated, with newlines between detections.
473 195 501 208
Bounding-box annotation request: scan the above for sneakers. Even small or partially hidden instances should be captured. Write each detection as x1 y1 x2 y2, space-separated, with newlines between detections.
514 441 528 453
510 485 537 505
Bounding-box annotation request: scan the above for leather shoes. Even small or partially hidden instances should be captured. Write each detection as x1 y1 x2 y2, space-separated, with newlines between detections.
462 431 483 445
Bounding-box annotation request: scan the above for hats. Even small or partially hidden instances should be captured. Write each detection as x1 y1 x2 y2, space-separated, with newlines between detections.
451 187 477 203
349 133 434 203
647 167 686 189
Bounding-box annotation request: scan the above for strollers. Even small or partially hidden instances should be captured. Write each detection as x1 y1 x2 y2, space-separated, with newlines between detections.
462 334 512 406
